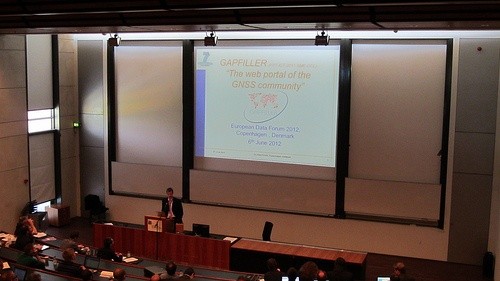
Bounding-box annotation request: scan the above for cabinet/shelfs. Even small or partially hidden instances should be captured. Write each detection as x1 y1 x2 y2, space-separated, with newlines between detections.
46 204 70 226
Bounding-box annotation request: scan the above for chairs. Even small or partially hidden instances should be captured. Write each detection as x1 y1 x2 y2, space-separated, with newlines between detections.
262 221 273 241
193 223 209 233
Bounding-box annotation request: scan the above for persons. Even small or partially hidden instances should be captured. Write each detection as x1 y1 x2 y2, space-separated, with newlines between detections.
0 215 417 281
161 187 183 230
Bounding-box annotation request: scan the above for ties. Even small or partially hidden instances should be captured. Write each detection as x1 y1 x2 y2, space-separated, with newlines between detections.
168 200 172 217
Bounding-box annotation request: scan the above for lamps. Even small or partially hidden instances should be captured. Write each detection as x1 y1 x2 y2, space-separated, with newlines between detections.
73 122 81 130
107 34 120 46
315 31 330 45
204 33 218 46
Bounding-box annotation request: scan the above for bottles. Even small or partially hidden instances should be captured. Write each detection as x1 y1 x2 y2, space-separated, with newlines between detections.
52 256 58 267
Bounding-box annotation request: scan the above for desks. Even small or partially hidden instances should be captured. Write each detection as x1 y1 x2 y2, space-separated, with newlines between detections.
0 229 368 281
